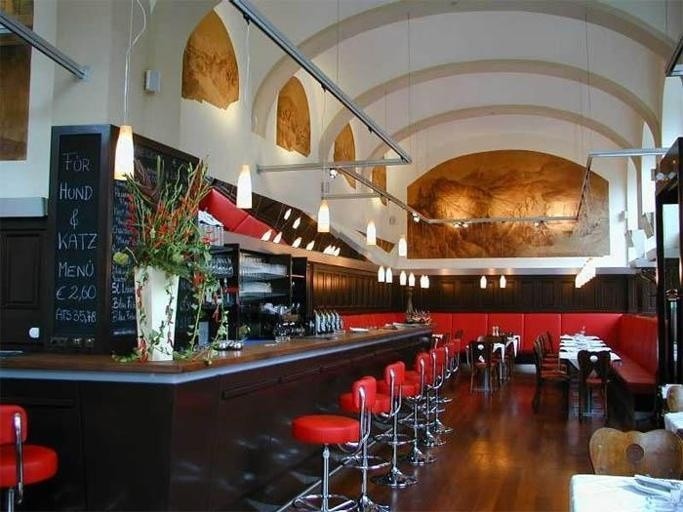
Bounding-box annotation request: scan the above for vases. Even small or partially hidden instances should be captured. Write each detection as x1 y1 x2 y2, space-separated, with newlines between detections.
134 264 179 361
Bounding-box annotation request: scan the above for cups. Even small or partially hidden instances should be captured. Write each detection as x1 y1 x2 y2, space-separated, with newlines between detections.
202 253 289 277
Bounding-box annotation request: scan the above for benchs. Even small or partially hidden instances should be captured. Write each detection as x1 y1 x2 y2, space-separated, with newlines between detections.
424 312 620 365
604 312 659 421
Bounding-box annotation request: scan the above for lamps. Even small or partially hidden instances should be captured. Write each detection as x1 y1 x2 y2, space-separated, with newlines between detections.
366 166 376 245
399 208 407 256
318 85 330 232
375 212 603 292
236 20 252 209
115 1 135 182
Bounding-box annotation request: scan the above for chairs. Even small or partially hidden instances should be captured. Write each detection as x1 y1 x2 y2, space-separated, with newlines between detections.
279 335 464 511
0 403 60 511
467 323 519 395
568 383 683 512
532 324 626 423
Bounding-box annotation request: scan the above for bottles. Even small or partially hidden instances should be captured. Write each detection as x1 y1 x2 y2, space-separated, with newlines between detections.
314 308 341 334
491 326 499 337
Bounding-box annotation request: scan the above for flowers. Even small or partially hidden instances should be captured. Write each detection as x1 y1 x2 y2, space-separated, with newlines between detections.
117 150 226 364
